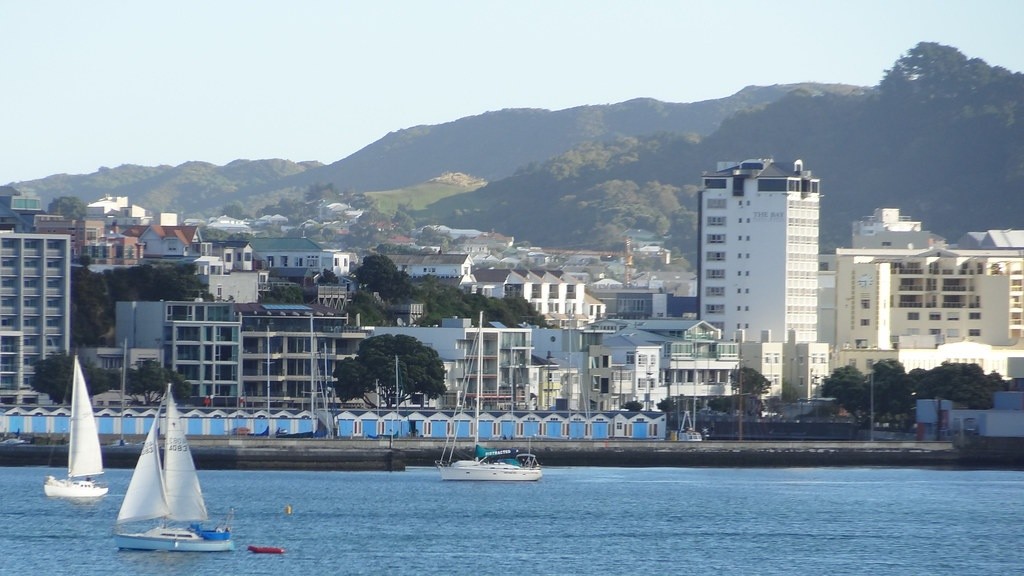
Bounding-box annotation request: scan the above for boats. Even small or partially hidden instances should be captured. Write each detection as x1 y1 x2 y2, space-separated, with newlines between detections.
246 545 283 553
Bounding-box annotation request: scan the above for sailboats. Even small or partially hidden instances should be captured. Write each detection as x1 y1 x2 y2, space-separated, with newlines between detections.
112 381 235 551
671 400 703 441
43 352 109 499
434 309 543 481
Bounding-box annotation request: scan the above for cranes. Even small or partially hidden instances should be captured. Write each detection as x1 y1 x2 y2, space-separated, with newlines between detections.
528 237 672 287
